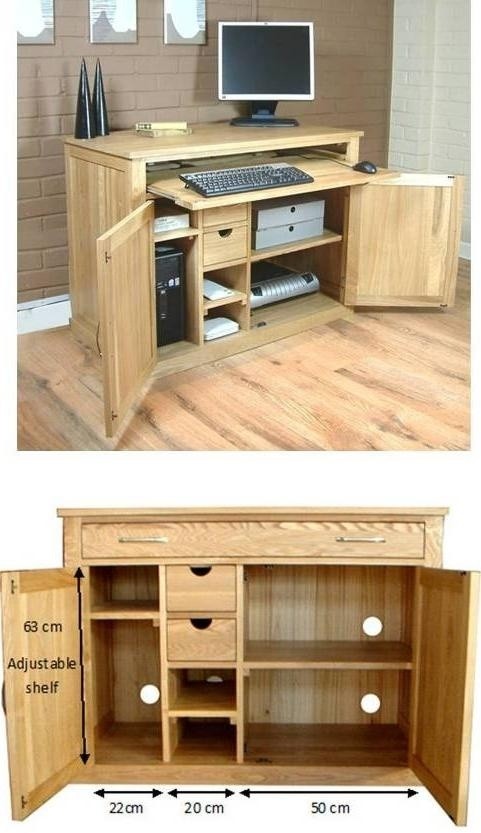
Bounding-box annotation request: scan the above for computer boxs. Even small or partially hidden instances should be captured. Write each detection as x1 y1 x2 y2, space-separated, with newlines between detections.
155 243 186 347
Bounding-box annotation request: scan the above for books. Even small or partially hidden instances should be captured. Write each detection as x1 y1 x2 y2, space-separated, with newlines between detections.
137 122 192 138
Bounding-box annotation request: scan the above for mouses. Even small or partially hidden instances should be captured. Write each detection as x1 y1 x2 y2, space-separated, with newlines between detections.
353 160 377 174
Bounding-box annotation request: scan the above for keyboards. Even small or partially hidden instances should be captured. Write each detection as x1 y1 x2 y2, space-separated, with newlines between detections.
179 162 313 196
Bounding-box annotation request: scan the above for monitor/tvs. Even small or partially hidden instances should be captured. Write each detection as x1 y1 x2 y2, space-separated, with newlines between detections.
218 21 315 127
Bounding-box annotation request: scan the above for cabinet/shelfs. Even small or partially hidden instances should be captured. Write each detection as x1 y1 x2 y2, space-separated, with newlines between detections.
64 125 468 438
1 505 481 827
